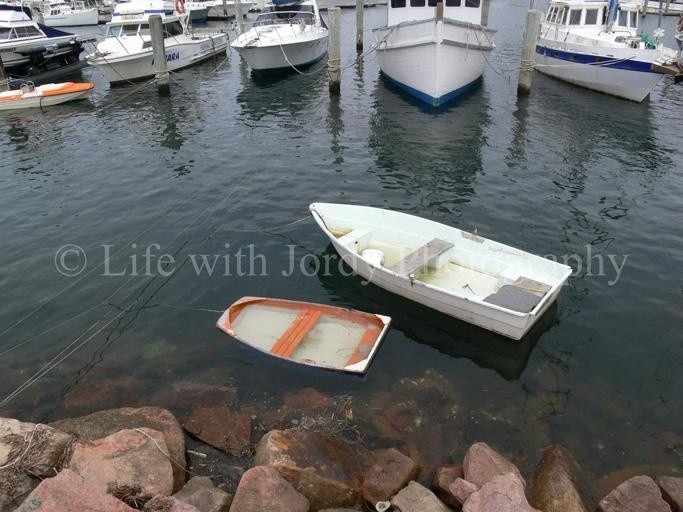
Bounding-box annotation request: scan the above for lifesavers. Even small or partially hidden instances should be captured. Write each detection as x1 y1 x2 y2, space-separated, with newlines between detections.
175 0 185 14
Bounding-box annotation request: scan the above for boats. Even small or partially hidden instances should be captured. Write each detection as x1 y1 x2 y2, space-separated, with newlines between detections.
532 0 677 102
1 81 95 110
85 27 230 83
307 201 575 342
375 1 496 107
0 0 267 26
229 0 328 71
0 20 97 89
